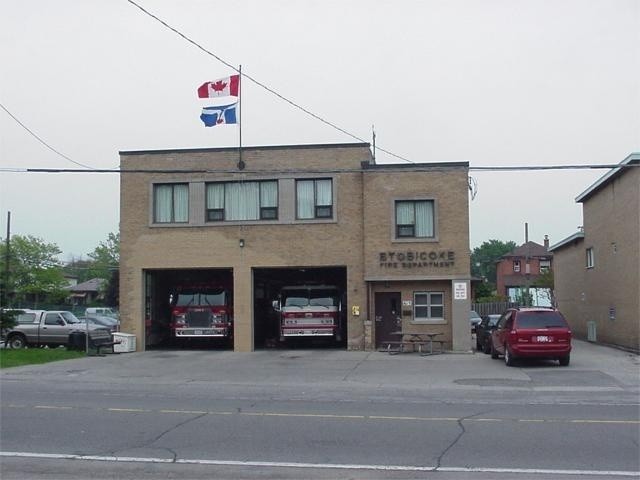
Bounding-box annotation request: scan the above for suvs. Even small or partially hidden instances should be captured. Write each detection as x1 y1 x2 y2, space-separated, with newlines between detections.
490 306 575 368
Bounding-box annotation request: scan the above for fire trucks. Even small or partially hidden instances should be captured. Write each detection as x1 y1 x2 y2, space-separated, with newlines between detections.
270 281 343 349
166 278 233 348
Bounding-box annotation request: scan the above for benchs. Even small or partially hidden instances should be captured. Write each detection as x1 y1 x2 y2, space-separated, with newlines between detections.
87 328 121 352
381 337 446 354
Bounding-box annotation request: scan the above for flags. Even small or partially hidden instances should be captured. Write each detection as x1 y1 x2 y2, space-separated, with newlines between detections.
200 100 240 128
197 73 241 98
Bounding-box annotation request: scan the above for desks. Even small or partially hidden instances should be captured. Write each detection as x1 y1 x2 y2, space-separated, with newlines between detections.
390 331 445 356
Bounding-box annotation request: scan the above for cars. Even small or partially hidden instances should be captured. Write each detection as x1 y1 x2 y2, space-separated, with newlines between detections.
471 310 484 334
474 312 503 354
1 305 121 353
